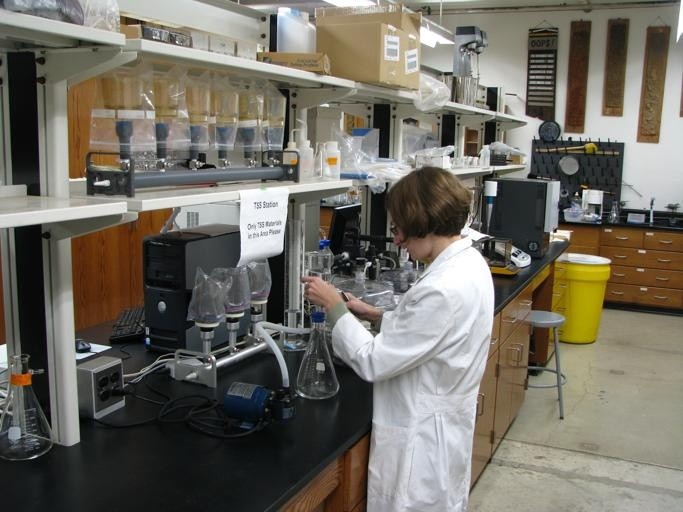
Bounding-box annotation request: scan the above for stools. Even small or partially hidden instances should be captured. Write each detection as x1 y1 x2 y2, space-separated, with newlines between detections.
526 310 567 421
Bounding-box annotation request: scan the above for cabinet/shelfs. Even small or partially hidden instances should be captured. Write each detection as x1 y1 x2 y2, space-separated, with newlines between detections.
355 82 495 261
2 10 138 448
494 110 525 178
470 284 531 497
71 36 355 340
599 228 683 312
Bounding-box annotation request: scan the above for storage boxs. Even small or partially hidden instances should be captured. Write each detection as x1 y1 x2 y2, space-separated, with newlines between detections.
314 11 426 90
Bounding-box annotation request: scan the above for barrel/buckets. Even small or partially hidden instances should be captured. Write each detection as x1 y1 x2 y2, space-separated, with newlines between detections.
549 252 613 344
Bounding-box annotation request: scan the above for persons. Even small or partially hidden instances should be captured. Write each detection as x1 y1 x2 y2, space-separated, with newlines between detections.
300 165 495 512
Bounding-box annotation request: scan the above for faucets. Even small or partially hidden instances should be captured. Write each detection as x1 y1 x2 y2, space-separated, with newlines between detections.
648 196 655 222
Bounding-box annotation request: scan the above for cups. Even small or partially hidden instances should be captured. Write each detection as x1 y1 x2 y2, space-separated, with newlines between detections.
436 75 455 102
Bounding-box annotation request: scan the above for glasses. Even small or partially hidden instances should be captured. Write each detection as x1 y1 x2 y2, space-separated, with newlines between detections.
390 224 398 233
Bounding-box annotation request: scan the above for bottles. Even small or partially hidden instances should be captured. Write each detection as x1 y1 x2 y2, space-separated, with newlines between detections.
294 310 340 399
481 144 490 169
0 354 54 461
282 119 341 182
308 253 331 285
609 201 620 223
571 192 582 212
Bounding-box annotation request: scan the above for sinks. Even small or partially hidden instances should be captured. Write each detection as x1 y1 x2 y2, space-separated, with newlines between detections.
651 216 682 228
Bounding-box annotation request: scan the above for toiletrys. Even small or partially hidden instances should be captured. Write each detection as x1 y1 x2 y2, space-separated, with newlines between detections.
570 192 582 211
606 196 621 224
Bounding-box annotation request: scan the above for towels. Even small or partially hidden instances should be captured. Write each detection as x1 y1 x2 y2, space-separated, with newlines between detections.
626 212 647 224
